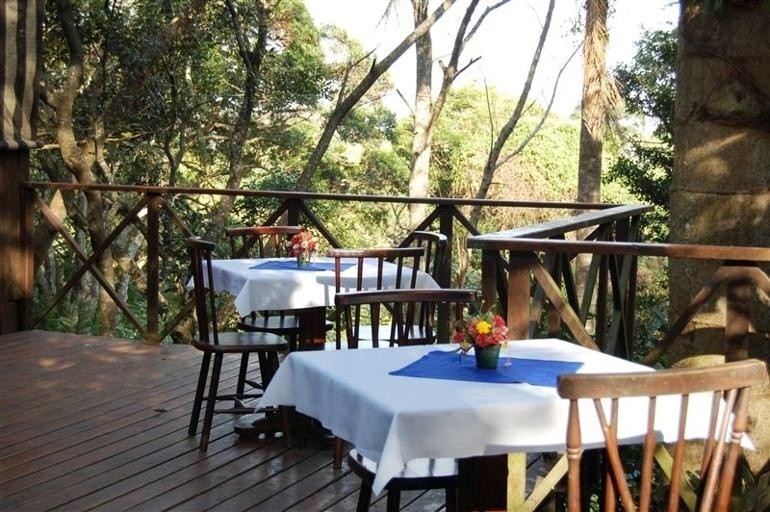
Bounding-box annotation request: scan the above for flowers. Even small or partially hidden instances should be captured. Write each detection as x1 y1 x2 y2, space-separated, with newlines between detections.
451 302 513 365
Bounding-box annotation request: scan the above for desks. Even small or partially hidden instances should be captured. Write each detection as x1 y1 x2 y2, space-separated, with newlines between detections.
191 253 448 351
252 335 759 512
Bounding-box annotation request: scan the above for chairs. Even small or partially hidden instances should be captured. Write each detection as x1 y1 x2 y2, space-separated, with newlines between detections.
324 246 428 349
355 228 450 347
184 234 301 454
223 225 336 416
553 354 767 512
330 286 483 510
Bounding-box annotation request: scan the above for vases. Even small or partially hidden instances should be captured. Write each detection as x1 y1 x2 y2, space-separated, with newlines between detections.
470 343 503 371
